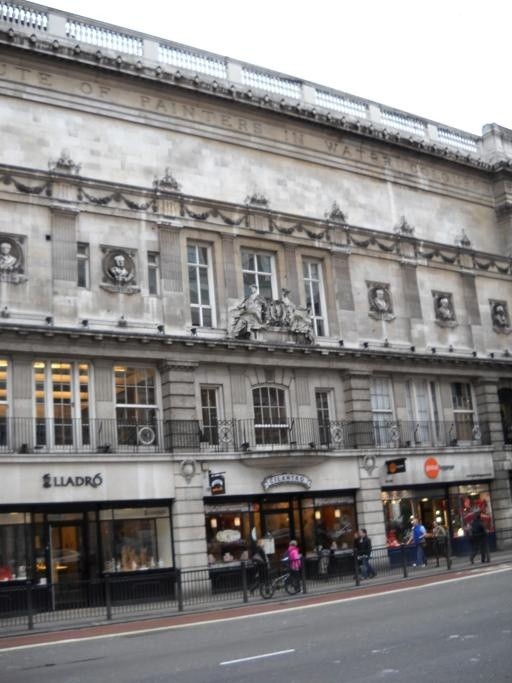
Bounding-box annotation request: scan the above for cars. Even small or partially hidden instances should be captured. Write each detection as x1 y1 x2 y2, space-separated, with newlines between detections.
269 526 300 546
208 535 249 548
333 523 353 538
35 547 79 565
115 537 141 557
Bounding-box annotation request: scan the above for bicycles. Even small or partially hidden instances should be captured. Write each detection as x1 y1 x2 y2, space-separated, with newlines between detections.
259 557 301 601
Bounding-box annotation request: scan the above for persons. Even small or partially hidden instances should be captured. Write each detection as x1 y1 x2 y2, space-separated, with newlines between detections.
109 254 129 281
436 297 452 320
493 305 510 328
0 242 17 272
288 540 303 592
279 287 312 332
469 509 487 564
352 528 377 581
231 284 264 338
372 289 388 312
406 515 452 569
